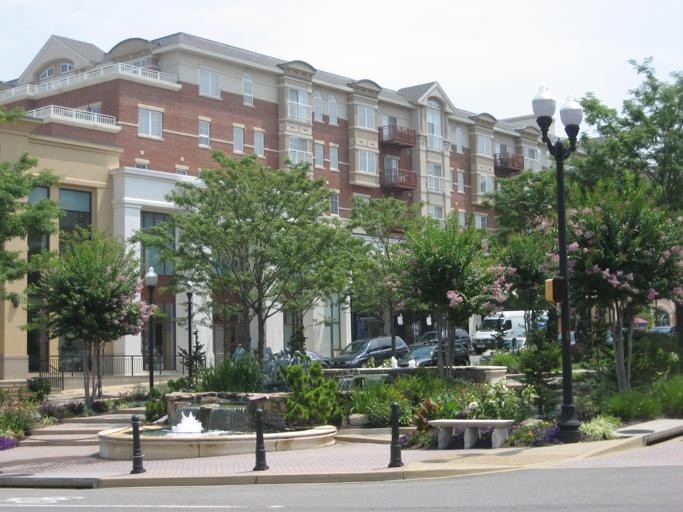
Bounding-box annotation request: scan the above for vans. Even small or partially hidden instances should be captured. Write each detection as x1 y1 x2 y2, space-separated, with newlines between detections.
472 310 548 355
331 335 408 370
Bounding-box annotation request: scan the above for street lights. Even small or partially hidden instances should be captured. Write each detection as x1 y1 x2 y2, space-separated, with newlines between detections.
183 277 197 384
531 84 584 442
143 266 159 388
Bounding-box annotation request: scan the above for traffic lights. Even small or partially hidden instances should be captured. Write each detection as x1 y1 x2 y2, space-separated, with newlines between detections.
544 277 564 302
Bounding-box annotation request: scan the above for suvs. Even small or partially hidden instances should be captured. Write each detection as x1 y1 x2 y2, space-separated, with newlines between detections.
398 344 470 368
408 329 471 356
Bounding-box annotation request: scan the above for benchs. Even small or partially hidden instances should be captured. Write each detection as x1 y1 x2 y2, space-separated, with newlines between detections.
426 418 518 452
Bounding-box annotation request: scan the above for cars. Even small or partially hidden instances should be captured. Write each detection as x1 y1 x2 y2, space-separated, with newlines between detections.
270 350 329 373
649 326 674 334
557 331 576 347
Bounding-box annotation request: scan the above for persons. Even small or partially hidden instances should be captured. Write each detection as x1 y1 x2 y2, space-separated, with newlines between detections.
233 343 245 366
